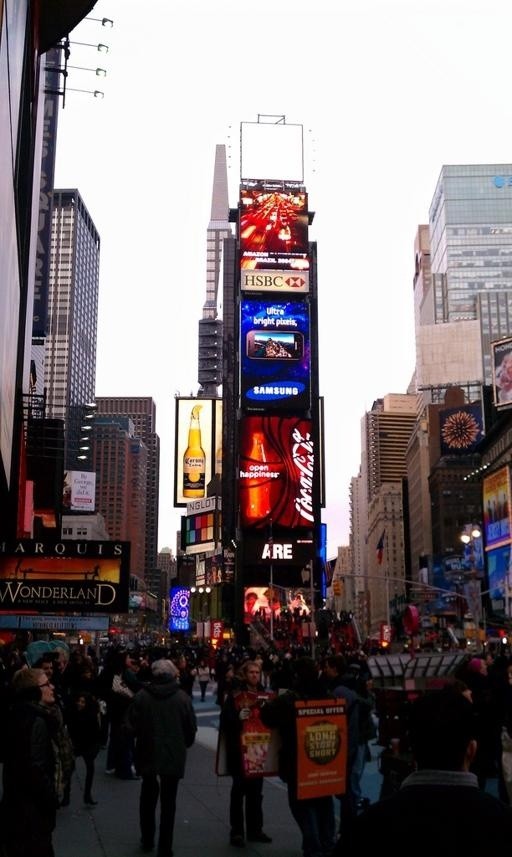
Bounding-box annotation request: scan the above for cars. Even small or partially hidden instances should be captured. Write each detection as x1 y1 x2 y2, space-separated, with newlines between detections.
363 630 511 661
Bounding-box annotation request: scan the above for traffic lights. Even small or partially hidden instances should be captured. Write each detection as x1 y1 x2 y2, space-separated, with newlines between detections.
331 579 344 596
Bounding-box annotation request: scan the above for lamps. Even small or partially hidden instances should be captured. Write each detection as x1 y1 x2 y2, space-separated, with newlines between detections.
43 16 114 108
458 511 482 544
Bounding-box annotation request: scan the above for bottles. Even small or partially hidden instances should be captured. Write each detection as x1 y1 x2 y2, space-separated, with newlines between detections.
247 430 275 521
183 409 210 498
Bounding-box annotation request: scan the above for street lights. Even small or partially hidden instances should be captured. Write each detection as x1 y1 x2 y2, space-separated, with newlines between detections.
189 585 212 622
459 522 483 652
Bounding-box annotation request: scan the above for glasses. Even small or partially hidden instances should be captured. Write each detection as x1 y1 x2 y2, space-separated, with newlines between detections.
40 679 50 687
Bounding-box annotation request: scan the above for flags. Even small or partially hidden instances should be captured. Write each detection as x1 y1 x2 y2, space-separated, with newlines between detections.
325 559 337 587
376 529 386 563
265 522 274 559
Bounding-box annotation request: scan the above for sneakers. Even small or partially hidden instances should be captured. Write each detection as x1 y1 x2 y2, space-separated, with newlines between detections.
140 830 272 856
62 764 141 807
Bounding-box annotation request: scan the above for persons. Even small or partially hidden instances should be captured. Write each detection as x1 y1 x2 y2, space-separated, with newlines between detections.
263 588 280 611
244 592 260 624
288 589 310 616
486 491 509 523
